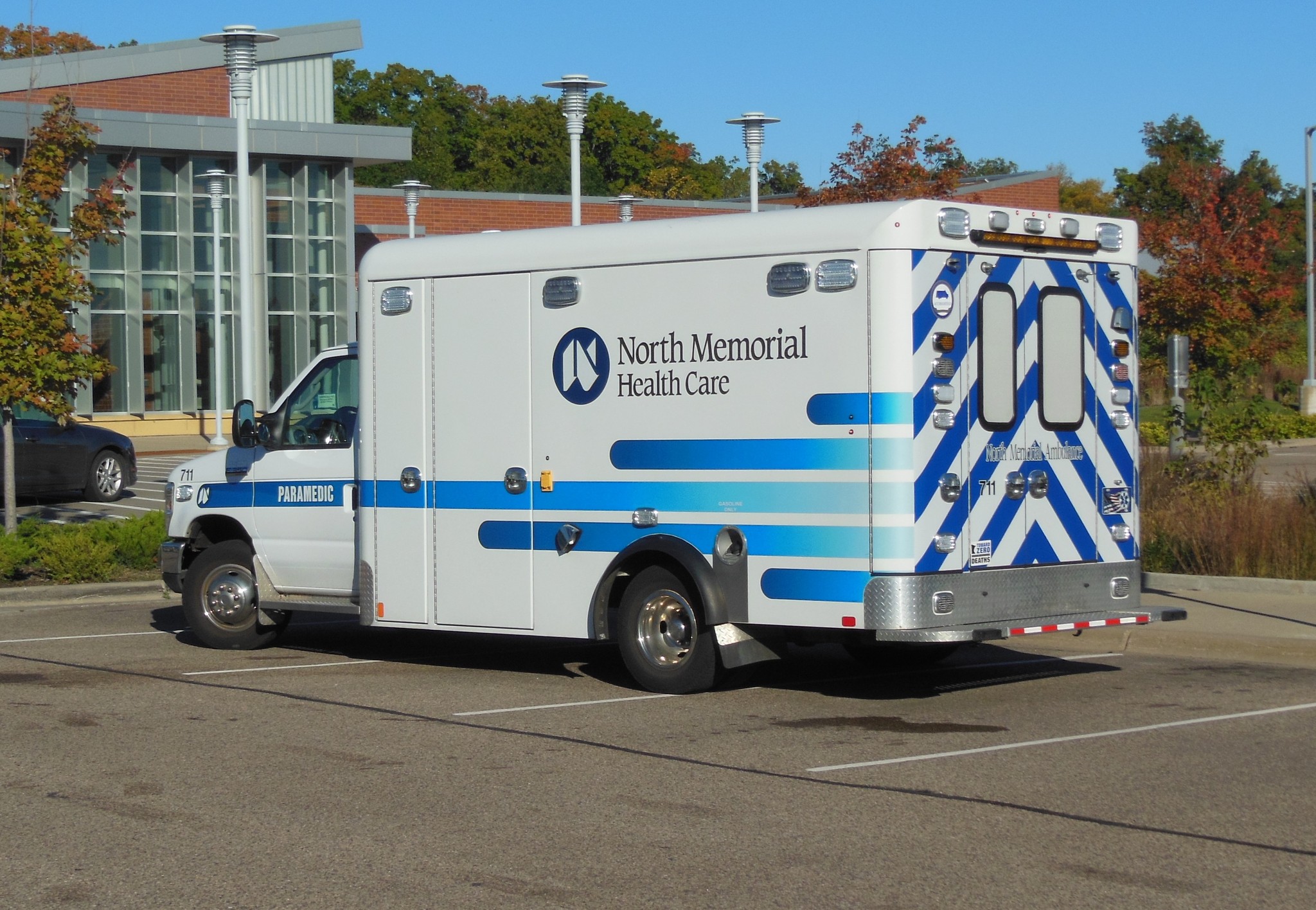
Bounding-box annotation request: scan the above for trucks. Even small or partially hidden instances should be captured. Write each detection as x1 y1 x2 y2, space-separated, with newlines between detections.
161 191 1192 696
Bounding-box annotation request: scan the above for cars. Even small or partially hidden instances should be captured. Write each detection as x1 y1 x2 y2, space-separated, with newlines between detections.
0 393 140 502
